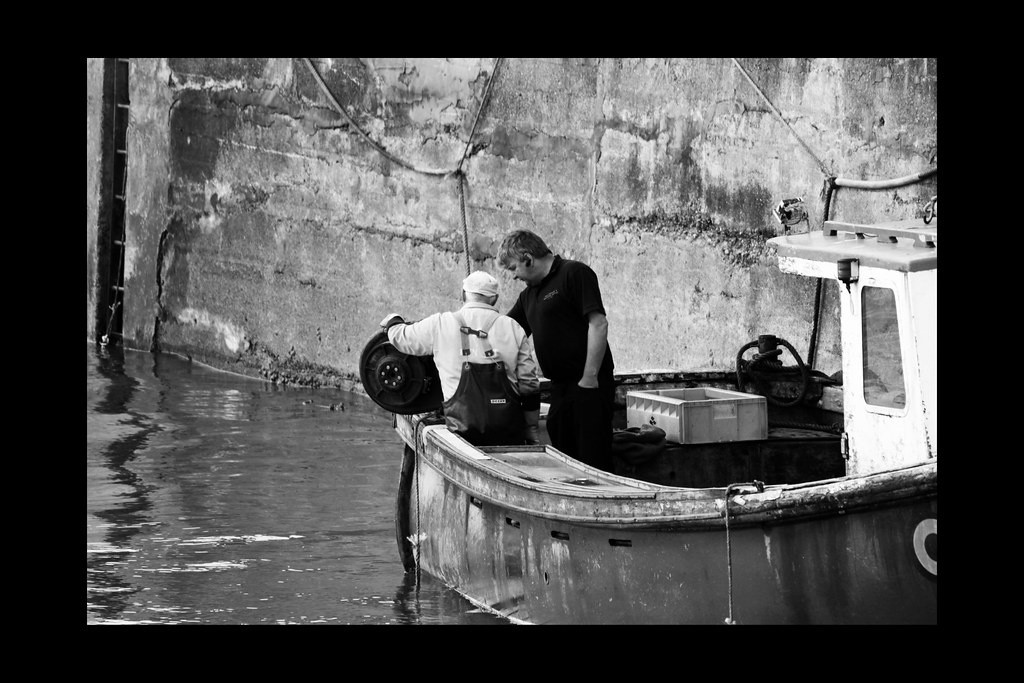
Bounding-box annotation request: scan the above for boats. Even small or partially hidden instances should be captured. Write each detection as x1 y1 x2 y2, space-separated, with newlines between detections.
368 193 938 625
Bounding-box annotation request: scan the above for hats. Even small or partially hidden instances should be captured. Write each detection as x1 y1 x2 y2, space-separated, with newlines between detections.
462 270 499 297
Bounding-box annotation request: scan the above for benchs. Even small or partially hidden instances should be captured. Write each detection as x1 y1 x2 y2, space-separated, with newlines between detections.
767 426 842 447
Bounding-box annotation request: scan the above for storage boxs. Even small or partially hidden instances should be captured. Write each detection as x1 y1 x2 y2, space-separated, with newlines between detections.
626 386 769 445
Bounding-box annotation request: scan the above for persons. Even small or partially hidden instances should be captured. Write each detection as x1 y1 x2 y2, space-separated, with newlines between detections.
497 226 618 475
380 269 544 444
